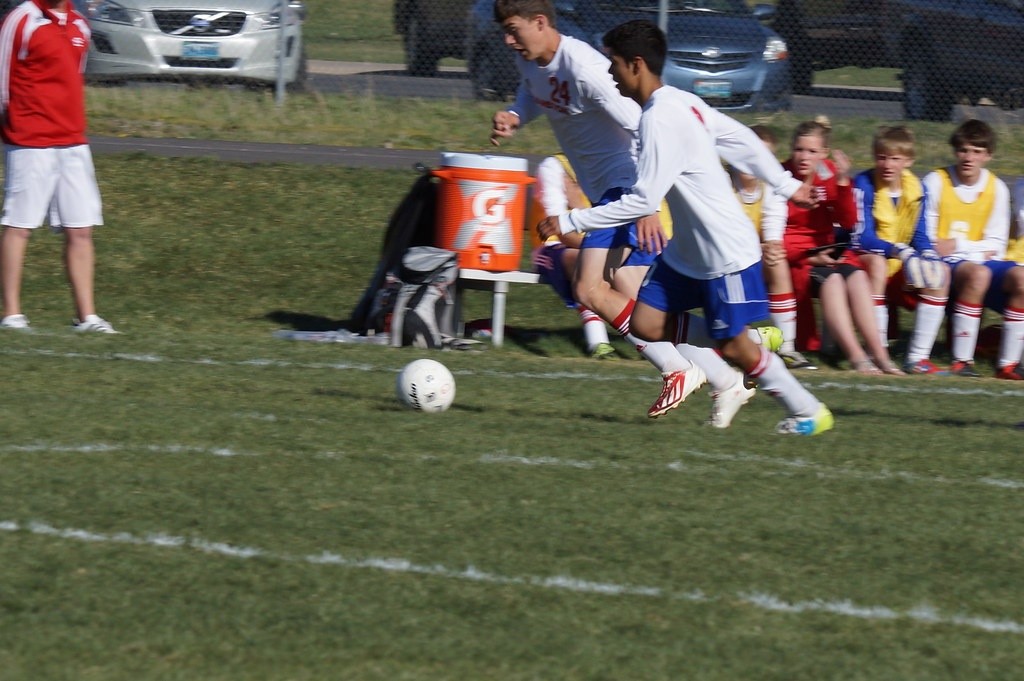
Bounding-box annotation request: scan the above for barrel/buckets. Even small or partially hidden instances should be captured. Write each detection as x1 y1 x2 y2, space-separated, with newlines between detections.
422 153 536 272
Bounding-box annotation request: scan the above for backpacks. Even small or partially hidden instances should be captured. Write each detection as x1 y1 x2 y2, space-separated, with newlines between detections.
389 245 487 354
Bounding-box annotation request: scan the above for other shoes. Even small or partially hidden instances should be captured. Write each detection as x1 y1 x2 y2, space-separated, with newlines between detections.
903 359 951 377
996 361 1024 380
950 360 983 377
850 359 905 375
776 352 819 370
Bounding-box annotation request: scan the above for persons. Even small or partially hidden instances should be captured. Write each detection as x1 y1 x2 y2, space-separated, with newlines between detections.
533 114 1024 381
533 20 833 436
490 0 754 428
0 0 126 334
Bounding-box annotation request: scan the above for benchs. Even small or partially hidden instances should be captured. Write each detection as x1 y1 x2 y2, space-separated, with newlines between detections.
459 268 546 348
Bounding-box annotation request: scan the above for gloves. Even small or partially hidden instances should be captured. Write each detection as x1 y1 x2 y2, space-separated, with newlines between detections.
890 242 945 289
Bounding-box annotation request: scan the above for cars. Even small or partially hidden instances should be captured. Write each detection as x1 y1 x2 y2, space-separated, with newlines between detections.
75 0 1024 125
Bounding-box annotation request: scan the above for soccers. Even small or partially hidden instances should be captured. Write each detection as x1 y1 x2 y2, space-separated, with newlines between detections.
399 359 456 413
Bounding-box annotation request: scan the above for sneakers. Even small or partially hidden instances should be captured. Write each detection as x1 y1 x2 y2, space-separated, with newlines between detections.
586 342 620 361
743 326 784 390
647 359 707 419
706 371 756 428
71 314 121 334
1 314 32 329
774 401 835 436
